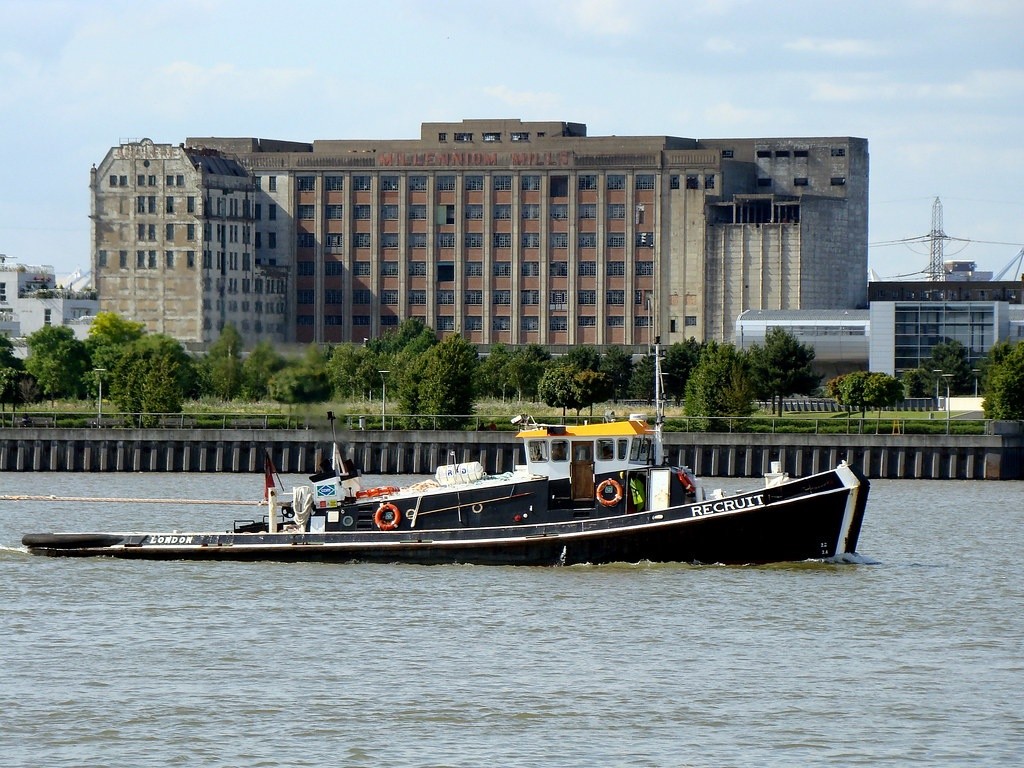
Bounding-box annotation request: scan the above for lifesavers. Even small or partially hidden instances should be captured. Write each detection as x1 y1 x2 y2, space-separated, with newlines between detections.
597 479 622 507
357 486 395 496
678 470 695 491
376 504 399 529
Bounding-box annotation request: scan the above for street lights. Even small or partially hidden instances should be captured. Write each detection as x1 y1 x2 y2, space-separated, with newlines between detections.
94 369 106 429
378 370 391 430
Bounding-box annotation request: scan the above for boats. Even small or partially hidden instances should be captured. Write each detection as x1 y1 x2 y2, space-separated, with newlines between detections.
19 333 872 573
